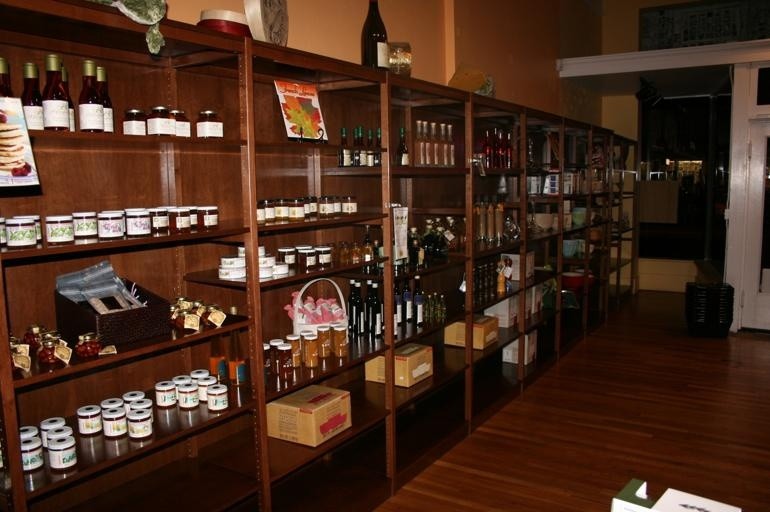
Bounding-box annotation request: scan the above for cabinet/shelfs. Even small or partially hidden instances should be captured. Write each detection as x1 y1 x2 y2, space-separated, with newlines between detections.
585 127 613 339
0 1 264 512
605 134 625 323
522 110 563 386
389 75 472 490
470 94 525 429
558 119 593 356
249 38 391 512
618 139 639 304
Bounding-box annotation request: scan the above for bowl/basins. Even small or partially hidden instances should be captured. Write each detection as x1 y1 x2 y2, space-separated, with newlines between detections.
560 272 584 292
572 212 585 225
582 244 594 254
533 214 553 230
563 240 579 256
587 273 594 286
575 240 585 252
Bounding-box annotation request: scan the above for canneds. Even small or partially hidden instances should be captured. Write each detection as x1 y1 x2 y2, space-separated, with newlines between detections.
298 249 317 271
19 417 78 474
332 196 341 216
36 339 63 366
154 369 229 414
45 216 74 243
5 219 37 250
125 211 152 236
146 208 168 234
12 215 42 245
23 324 41 349
167 208 191 234
170 296 221 330
170 110 191 137
145 105 176 136
97 213 124 240
257 196 318 225
277 247 296 265
196 206 219 231
71 212 97 238
122 109 146 135
179 206 198 228
390 42 412 77
195 110 223 137
75 333 102 358
101 210 126 235
315 246 331 267
318 196 334 219
77 390 154 442
0 217 6 244
342 196 358 216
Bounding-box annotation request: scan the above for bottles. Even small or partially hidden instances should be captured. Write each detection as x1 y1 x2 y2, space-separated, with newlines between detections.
270 339 283 375
327 239 374 263
394 276 448 339
423 121 433 165
393 216 466 275
473 193 505 242
78 60 104 133
447 124 456 165
19 63 43 130
276 344 293 380
440 124 449 166
360 0 390 69
429 122 441 165
228 304 247 386
0 57 14 97
375 128 381 167
355 126 366 167
42 54 70 132
301 330 313 362
286 335 300 367
483 127 512 169
329 322 341 351
349 128 358 166
337 128 354 167
61 67 76 132
303 335 318 368
209 337 226 381
396 128 409 166
317 325 331 357
96 66 114 132
264 344 271 378
333 326 347 358
376 225 384 270
362 225 373 275
414 120 425 165
347 279 384 338
366 129 375 167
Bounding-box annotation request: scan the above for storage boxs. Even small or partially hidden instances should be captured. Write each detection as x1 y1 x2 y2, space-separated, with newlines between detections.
500 249 535 282
524 287 529 320
532 285 544 314
365 343 433 388
266 385 352 448
502 330 538 365
484 293 519 329
444 313 499 351
